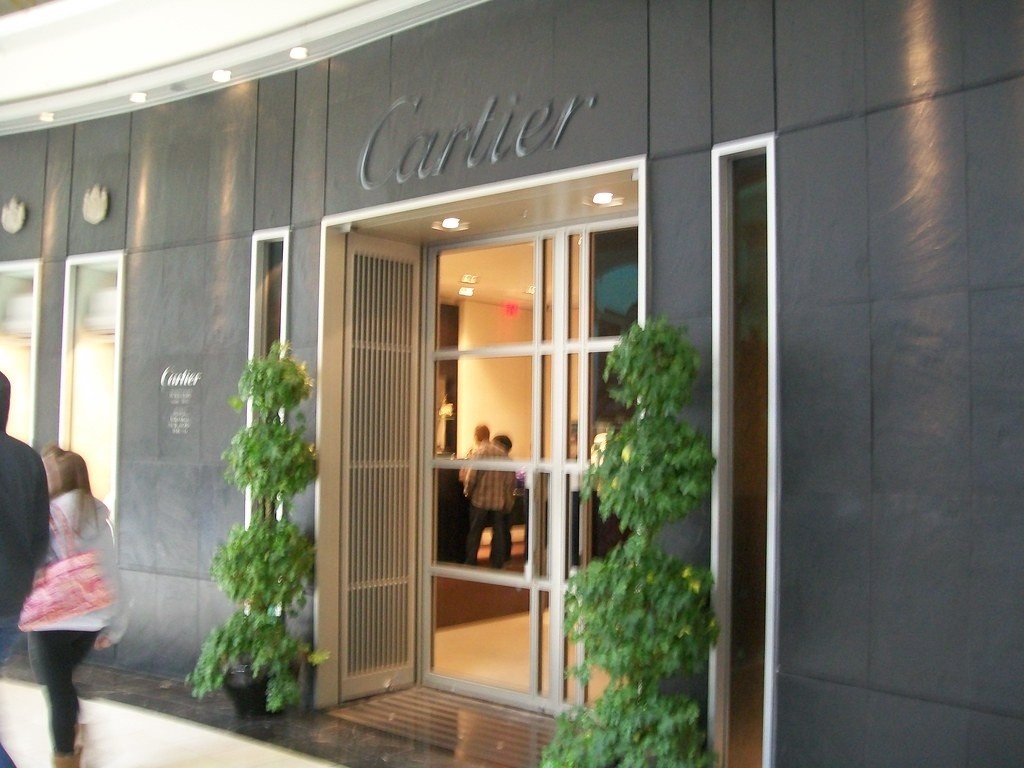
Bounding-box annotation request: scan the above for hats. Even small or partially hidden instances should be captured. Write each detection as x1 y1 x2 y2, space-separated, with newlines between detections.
494 435 512 448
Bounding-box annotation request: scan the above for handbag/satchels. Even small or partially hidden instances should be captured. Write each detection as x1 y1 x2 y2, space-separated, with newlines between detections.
18 502 115 632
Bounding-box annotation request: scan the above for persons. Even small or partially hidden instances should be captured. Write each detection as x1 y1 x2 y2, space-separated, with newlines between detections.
0 373 48 767
459 424 515 569
22 444 128 768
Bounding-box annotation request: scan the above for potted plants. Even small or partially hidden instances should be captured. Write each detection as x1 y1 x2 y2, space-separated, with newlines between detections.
185 339 328 718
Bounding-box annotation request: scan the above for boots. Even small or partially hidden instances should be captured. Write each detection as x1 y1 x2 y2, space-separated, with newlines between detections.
52 746 82 768
